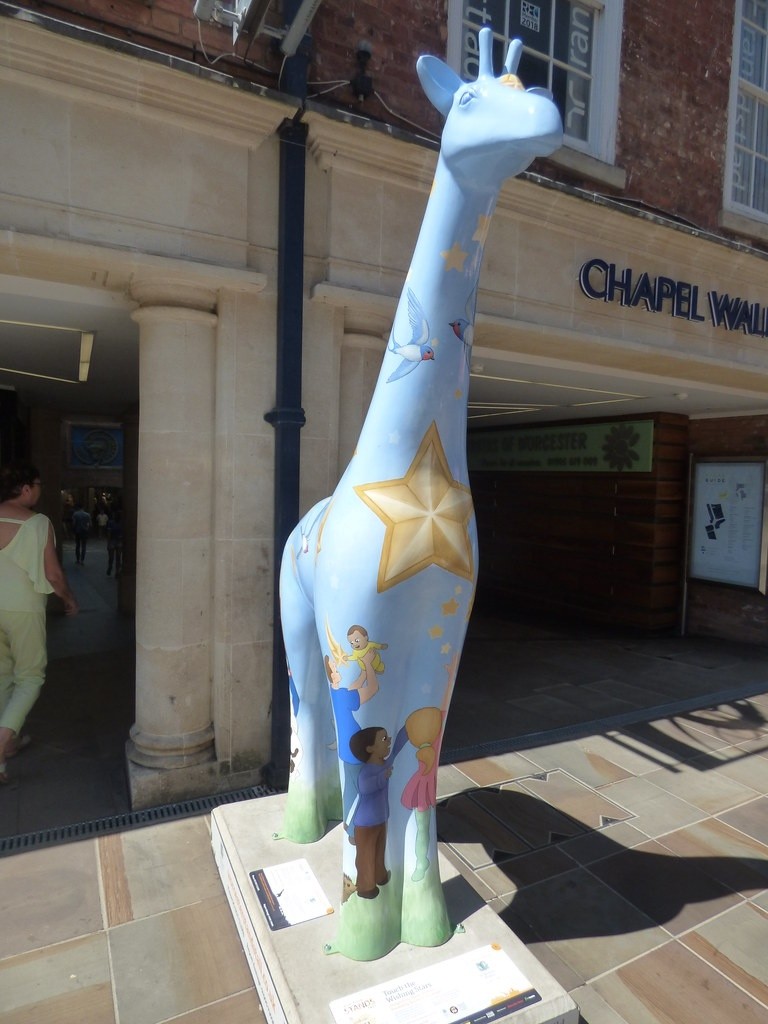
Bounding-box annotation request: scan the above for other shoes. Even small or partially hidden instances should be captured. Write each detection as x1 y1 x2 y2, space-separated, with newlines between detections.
107 564 113 576
4 735 31 758
0 763 8 785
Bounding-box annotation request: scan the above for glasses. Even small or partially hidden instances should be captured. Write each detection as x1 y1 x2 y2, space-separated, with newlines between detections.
31 480 44 489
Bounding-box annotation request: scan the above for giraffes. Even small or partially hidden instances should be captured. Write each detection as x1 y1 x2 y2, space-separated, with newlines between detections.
279 28 563 963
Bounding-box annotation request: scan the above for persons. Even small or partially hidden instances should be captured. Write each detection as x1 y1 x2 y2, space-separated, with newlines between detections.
106 511 123 575
96 509 109 539
0 459 79 784
70 500 94 566
